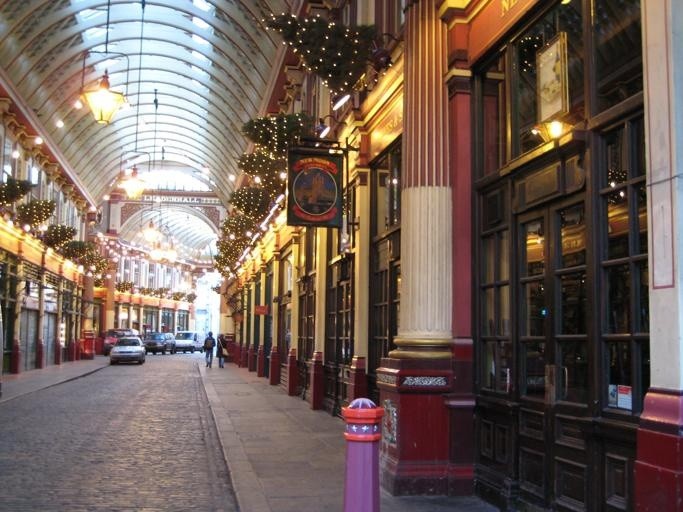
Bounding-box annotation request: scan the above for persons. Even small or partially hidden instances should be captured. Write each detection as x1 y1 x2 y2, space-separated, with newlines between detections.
204 332 215 368
216 334 226 368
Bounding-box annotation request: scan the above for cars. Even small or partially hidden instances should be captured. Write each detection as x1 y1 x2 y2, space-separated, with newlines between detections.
105 329 203 364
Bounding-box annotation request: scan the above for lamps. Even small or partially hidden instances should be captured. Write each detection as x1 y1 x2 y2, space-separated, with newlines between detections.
368 30 403 71
311 85 373 143
78 1 131 129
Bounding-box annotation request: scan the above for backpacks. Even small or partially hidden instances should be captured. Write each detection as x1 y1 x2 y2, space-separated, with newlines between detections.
206 337 213 350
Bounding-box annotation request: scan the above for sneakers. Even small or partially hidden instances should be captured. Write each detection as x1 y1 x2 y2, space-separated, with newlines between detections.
206 363 224 368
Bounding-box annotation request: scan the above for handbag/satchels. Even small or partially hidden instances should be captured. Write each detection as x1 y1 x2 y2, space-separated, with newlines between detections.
222 348 229 357
